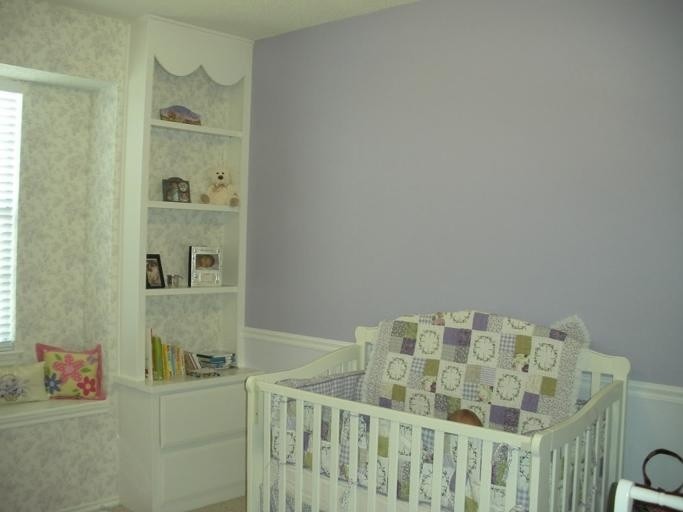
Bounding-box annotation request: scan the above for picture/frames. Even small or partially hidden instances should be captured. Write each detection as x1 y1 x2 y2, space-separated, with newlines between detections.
190 246 223 286
146 254 165 288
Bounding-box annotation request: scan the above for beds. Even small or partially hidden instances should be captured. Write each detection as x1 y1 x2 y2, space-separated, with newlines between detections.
244 324 630 512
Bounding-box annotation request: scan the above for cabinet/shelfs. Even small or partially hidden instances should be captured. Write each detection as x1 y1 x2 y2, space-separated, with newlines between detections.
114 369 264 512
146 118 242 296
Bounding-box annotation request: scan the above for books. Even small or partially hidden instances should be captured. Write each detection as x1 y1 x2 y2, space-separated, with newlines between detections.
146 327 236 386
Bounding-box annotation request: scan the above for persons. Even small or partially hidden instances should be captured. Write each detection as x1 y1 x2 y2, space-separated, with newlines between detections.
196 254 215 267
447 409 482 427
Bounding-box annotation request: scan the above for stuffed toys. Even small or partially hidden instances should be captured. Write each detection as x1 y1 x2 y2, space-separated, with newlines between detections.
201 167 240 207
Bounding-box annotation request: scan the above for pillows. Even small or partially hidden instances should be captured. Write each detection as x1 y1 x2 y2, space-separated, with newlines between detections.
34 343 107 400
0 361 50 402
361 310 589 439
